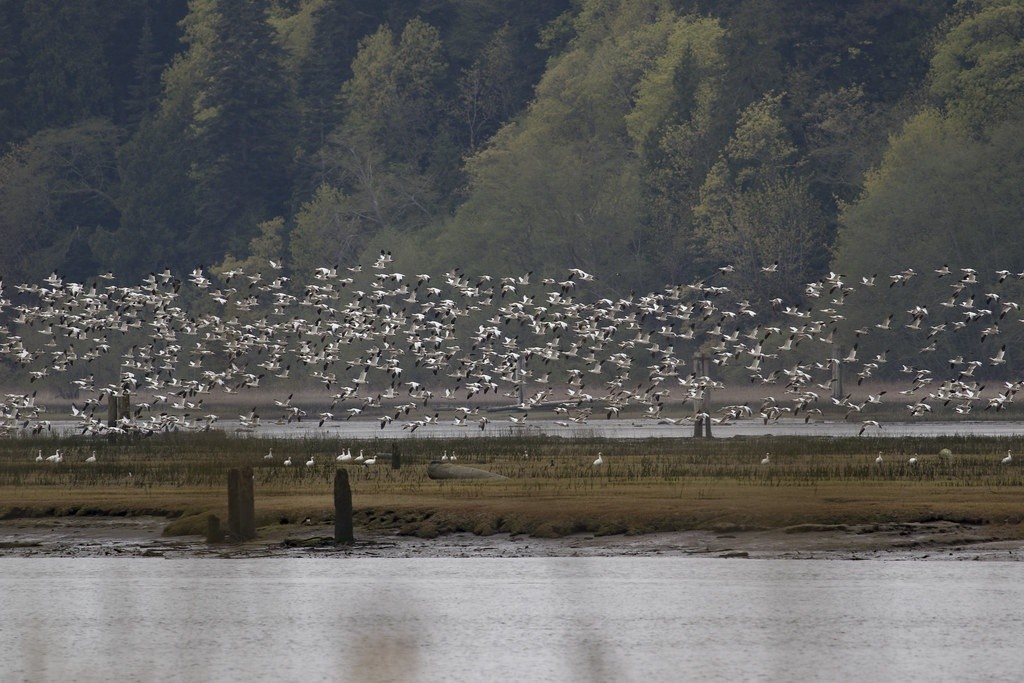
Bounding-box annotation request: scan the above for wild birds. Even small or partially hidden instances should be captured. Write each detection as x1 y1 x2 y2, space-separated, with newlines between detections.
36 447 1013 466
0 249 1024 436
